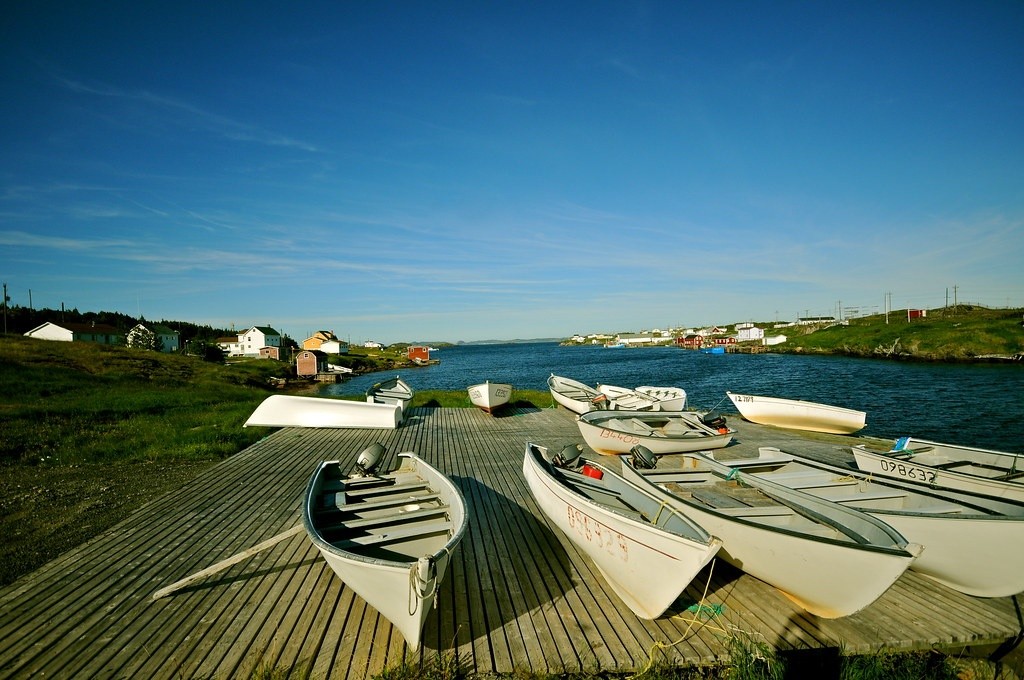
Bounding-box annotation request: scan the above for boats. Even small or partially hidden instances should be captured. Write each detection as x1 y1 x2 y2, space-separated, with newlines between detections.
365 374 415 412
575 409 739 456
242 394 405 431
851 436 1024 507
726 391 868 435
466 380 512 416
635 386 688 412
700 347 725 354
428 348 440 352
607 344 626 349
618 444 926 620
698 447 1024 598
596 382 661 411
522 442 724 621
547 372 616 415
302 443 470 653
